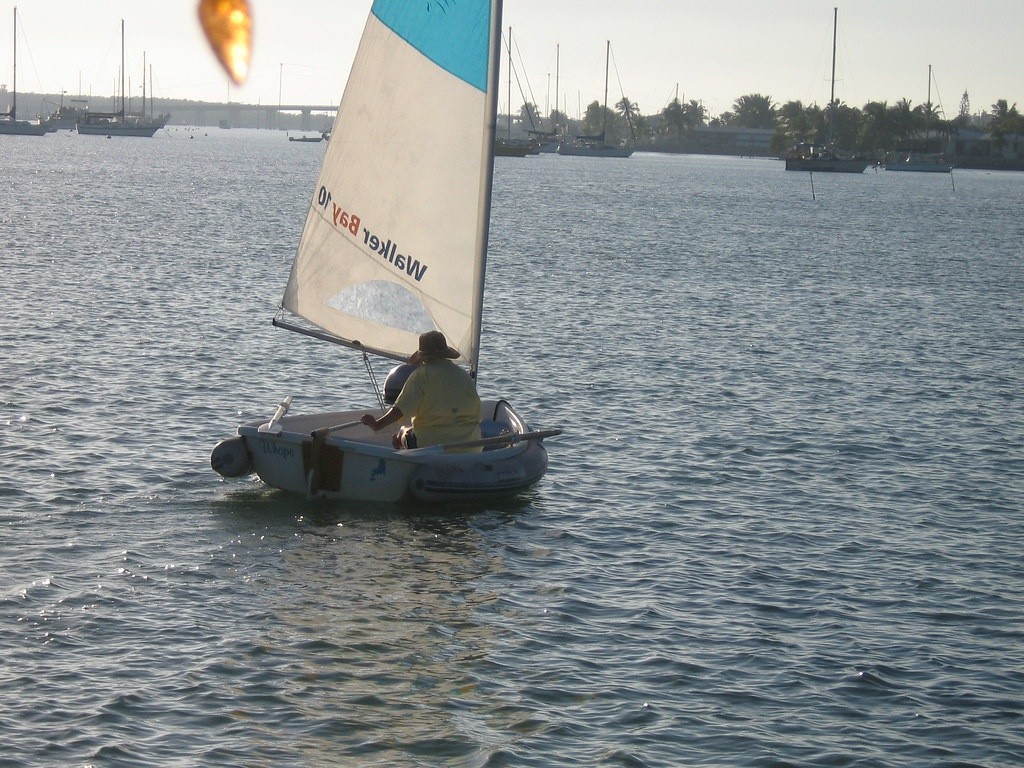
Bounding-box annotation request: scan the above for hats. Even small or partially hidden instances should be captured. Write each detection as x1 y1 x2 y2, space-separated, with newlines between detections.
409 330 460 366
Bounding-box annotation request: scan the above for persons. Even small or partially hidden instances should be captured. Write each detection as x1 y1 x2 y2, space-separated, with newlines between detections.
360 332 483 454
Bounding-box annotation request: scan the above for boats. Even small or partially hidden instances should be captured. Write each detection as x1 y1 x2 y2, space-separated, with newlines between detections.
288 129 324 143
322 127 332 142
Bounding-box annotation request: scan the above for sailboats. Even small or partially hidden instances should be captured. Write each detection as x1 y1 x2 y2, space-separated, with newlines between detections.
555 40 640 158
880 61 957 174
218 80 231 130
784 7 871 174
0 3 171 138
492 25 567 158
211 0 561 516
